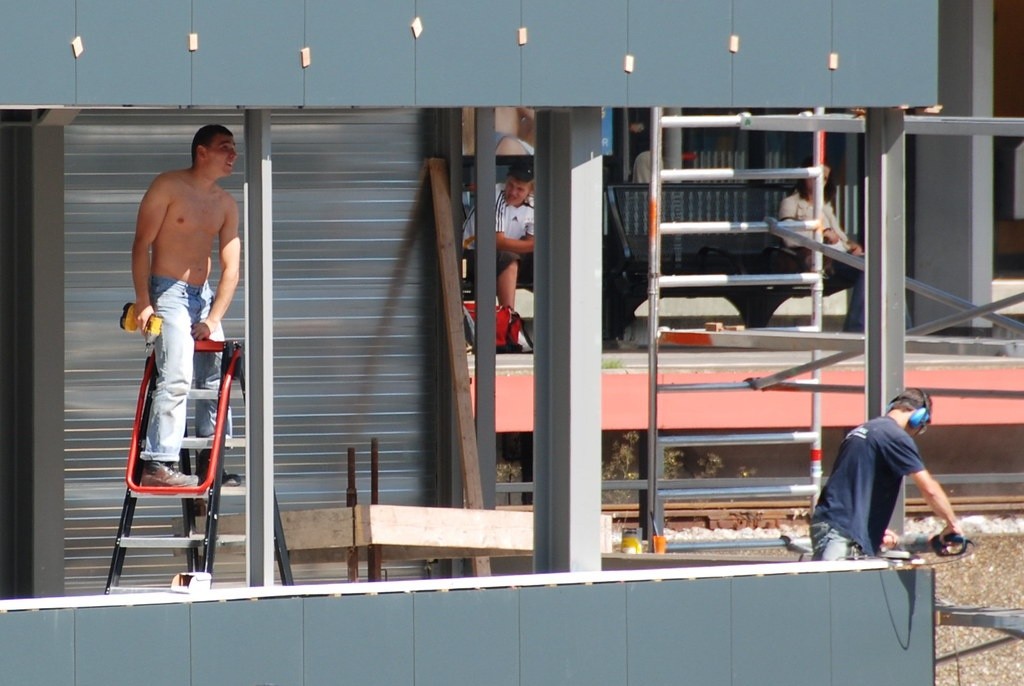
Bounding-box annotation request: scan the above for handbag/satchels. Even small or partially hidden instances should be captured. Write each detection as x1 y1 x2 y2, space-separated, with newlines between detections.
463 302 536 356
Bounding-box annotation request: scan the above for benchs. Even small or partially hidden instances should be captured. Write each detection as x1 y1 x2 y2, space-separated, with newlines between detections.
606 180 864 350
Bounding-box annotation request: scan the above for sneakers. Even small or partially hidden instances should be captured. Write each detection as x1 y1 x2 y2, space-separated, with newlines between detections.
195 465 240 487
141 459 200 488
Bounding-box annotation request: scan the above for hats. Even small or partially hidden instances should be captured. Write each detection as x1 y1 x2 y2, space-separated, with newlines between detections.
507 162 534 183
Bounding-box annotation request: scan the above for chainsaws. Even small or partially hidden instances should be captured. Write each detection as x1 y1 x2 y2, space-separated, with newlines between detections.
878 532 977 563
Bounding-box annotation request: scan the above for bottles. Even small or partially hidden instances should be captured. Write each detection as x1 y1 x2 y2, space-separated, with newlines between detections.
621 527 642 554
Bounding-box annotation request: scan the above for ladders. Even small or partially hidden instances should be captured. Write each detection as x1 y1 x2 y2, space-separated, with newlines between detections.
104 338 294 594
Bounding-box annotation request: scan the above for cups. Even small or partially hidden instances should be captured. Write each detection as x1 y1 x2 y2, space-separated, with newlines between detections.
654 536 667 554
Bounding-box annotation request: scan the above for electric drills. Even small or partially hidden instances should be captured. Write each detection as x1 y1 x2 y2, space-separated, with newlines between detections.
122 301 165 354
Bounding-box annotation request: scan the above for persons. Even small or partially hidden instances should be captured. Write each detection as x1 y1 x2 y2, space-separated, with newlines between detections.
463 164 535 312
131 125 241 487
633 142 667 184
780 157 865 334
811 388 963 562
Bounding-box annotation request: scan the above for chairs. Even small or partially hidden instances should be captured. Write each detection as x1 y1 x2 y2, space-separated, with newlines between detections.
457 176 538 353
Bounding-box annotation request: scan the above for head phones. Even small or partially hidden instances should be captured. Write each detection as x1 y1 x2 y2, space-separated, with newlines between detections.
887 387 930 429
